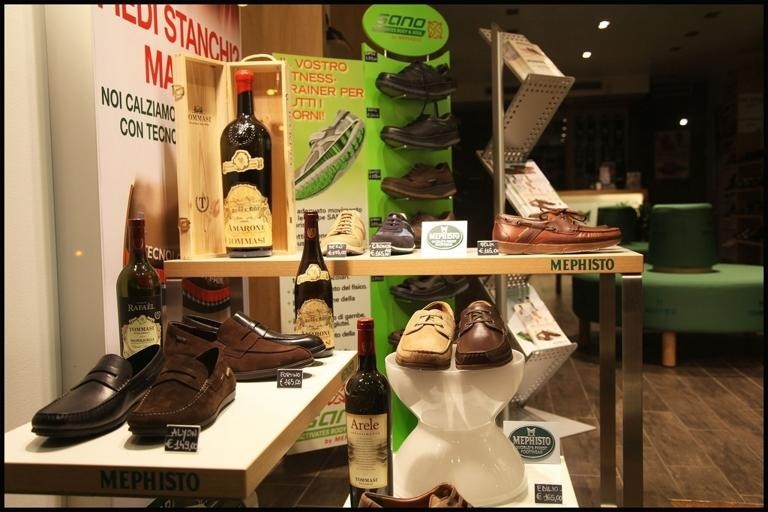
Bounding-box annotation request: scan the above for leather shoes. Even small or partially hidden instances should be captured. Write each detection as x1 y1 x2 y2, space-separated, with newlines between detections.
388 276 512 368
357 483 472 507
32 312 326 437
492 207 622 253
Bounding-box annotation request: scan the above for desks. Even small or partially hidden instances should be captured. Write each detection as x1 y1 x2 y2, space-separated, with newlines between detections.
154 244 645 510
3 348 358 509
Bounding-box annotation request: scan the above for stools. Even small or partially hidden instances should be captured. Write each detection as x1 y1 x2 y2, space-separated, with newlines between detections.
378 347 533 504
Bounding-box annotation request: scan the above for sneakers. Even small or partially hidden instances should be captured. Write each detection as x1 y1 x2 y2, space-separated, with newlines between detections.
294 109 364 199
318 210 455 254
373 62 461 200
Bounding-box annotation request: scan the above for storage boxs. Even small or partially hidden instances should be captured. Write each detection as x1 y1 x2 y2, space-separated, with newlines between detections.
167 48 300 260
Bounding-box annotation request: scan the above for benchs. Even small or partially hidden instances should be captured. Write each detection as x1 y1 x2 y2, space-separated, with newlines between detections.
571 243 764 367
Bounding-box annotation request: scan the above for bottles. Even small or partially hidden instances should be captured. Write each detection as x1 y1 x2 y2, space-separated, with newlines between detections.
125 118 183 331
218 71 274 259
116 218 165 358
291 212 335 357
340 316 393 507
